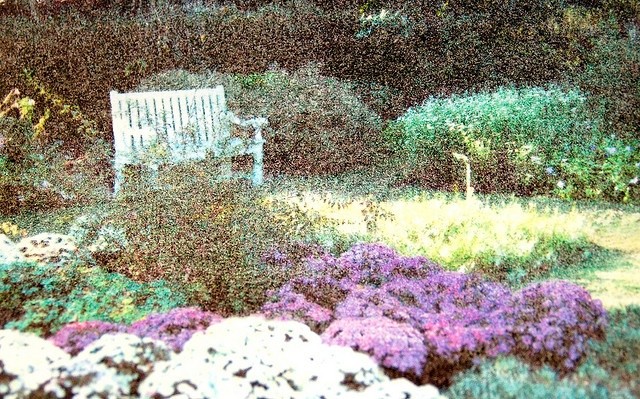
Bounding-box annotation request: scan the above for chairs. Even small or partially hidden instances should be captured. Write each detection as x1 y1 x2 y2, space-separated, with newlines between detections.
104 85 270 203
